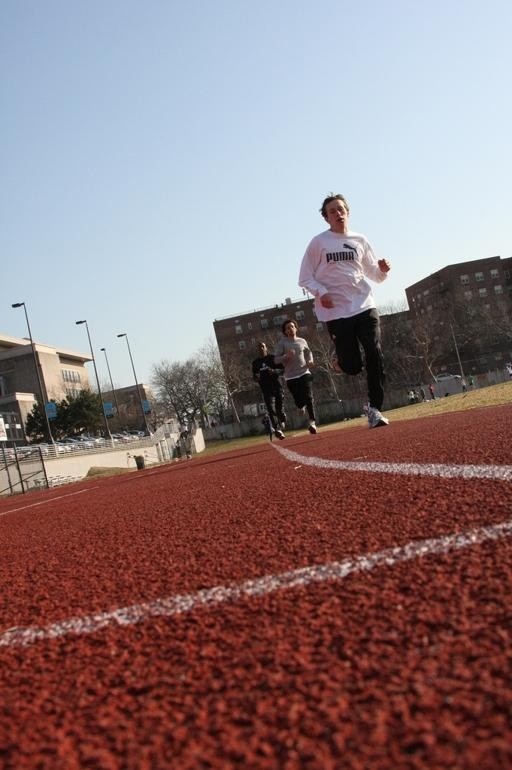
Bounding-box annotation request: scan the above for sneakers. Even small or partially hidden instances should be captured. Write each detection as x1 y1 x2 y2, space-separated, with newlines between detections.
307 423 318 434
368 408 390 428
274 423 287 441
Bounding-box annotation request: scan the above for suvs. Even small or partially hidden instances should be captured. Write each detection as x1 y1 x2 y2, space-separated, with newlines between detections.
436 372 462 383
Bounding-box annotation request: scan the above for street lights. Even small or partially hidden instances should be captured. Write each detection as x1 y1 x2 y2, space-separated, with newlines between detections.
76 319 150 447
12 303 60 459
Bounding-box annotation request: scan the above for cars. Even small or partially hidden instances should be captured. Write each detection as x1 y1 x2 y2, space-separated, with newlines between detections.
0 430 146 462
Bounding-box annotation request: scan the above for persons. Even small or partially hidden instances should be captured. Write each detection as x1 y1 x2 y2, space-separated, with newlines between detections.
251 342 289 440
260 411 272 435
273 320 319 435
298 192 392 428
406 375 474 404
